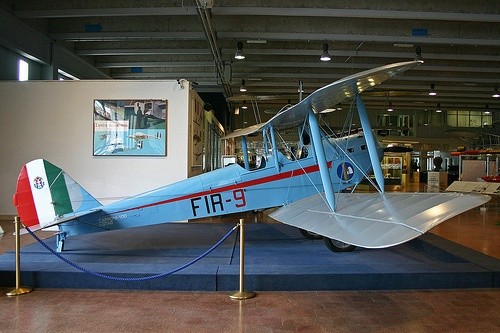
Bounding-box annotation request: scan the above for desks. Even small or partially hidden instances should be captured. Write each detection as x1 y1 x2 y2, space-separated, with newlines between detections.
451 149 499 181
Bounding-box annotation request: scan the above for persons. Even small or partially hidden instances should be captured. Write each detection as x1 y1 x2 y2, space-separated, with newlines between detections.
413 158 419 172
136 107 150 129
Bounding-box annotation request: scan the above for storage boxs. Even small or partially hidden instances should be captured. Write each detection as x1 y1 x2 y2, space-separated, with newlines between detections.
462 160 495 181
428 171 448 187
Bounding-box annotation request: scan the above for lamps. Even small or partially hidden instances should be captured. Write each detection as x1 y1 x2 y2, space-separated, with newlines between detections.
320 43 331 61
483 104 491 115
435 103 442 113
492 86 500 98
242 101 248 109
234 42 245 59
239 79 247 91
414 47 424 64
429 83 436 96
387 102 394 112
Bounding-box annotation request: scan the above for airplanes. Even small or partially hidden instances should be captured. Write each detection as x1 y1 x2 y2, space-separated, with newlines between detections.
13 59 492 253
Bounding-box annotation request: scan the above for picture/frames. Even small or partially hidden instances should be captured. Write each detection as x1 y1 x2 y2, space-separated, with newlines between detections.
92 99 167 157
